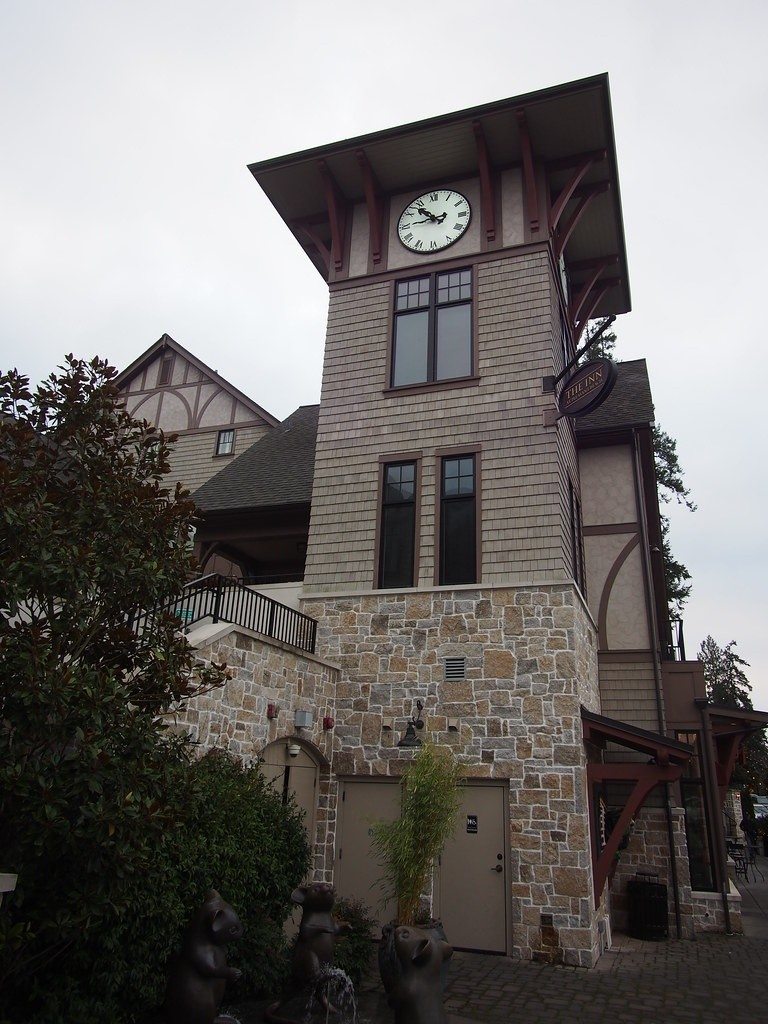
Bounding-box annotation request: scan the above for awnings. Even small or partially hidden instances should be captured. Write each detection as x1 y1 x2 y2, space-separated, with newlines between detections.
708 704 768 810
582 707 698 910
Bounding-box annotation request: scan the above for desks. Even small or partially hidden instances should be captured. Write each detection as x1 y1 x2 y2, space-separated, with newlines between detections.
730 855 747 880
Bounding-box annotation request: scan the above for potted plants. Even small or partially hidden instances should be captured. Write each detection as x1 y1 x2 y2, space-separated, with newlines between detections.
367 739 471 1010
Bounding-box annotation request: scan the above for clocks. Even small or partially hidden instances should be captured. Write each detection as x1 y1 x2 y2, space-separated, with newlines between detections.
397 189 472 255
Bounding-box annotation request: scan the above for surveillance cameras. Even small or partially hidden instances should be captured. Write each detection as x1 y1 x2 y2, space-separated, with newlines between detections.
289 745 300 757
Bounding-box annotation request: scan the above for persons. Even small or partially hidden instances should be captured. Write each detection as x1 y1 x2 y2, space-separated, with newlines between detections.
740 811 754 846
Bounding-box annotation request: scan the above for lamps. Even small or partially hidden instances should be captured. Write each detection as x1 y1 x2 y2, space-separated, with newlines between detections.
396 718 425 748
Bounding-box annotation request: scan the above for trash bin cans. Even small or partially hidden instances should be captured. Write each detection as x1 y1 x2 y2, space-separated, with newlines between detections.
626 872 669 942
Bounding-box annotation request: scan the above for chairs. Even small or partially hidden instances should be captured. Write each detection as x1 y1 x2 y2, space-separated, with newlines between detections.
730 843 765 883
